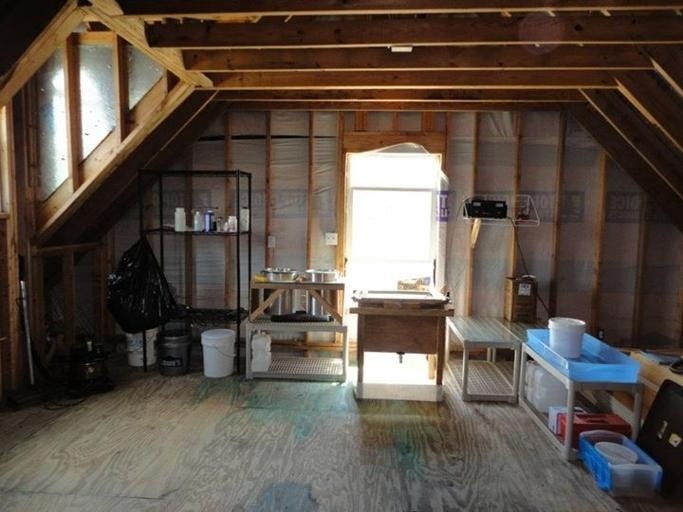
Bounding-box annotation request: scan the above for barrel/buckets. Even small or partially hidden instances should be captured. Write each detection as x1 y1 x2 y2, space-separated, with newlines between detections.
200 329 237 378
158 330 190 376
125 328 160 368
305 268 341 322
68 342 107 396
263 265 298 318
548 317 586 359
595 442 638 490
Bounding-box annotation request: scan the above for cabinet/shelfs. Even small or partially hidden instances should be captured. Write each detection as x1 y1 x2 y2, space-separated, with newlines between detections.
137 167 252 376
245 280 347 382
446 316 521 404
518 343 644 461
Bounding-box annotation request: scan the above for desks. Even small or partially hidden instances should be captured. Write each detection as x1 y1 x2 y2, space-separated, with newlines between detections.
350 305 454 401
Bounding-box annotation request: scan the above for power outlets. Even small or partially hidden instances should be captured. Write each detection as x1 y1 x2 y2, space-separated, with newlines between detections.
326 233 338 246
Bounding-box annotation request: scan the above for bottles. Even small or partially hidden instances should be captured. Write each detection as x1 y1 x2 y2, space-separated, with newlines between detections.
174 207 186 232
205 209 214 232
216 216 237 232
239 207 249 232
194 211 202 231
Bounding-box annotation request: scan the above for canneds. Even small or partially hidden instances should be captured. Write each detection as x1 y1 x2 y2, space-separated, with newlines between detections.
204 211 214 231
